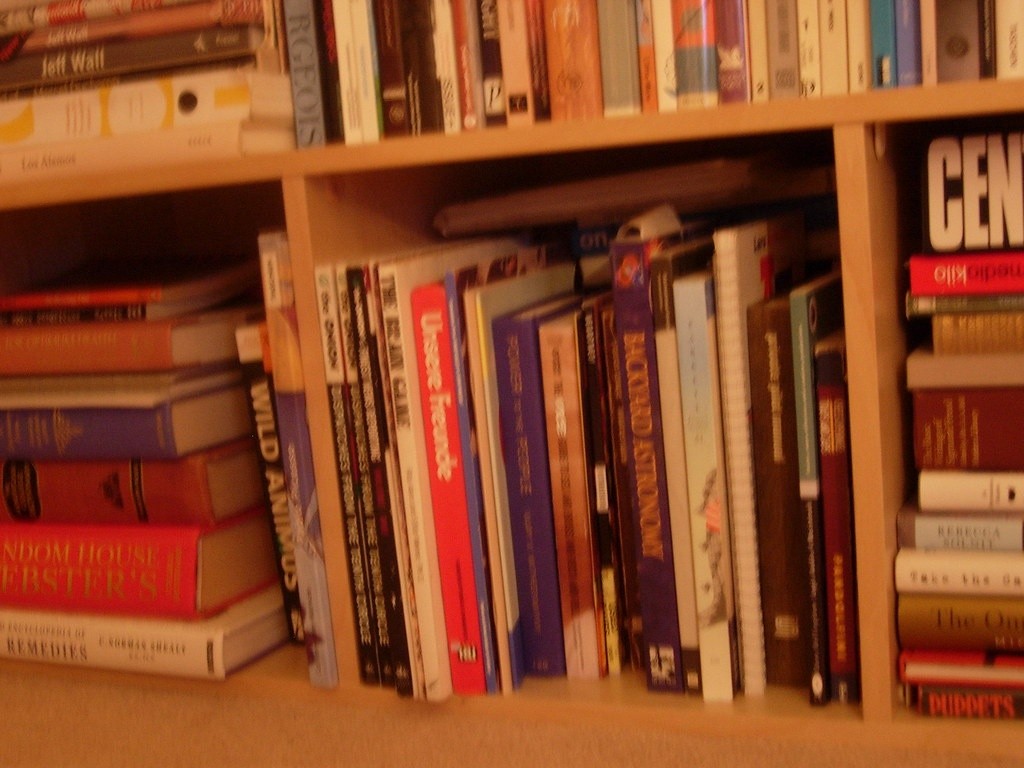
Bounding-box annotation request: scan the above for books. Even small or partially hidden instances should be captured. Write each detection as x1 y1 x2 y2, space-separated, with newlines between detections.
3 137 1024 721
0 1 1023 180
